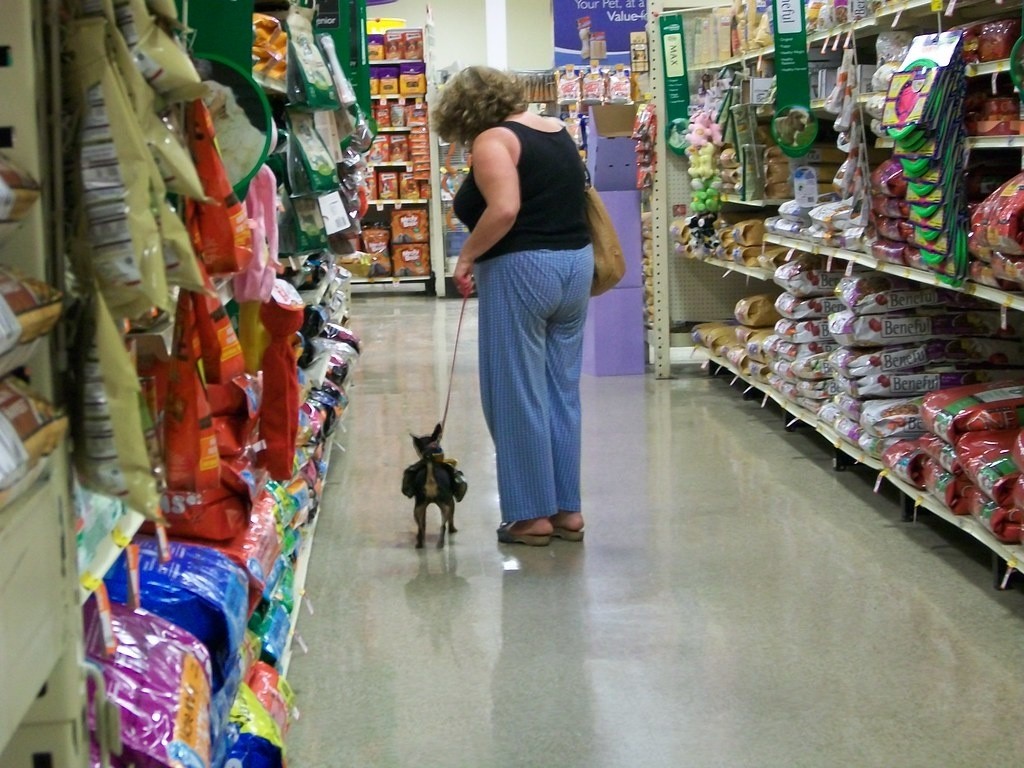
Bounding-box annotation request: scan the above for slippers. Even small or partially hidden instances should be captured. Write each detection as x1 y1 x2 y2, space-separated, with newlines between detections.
551 527 583 541
497 521 552 546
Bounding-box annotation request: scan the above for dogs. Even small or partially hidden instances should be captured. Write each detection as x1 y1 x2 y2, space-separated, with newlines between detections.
407 422 458 551
200 79 266 190
775 108 810 147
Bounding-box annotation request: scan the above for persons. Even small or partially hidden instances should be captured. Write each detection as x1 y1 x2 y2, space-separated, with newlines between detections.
431 66 594 546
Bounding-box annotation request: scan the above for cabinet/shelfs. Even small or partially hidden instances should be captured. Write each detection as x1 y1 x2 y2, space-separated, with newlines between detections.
351 26 432 296
0 0 91 768
646 0 1024 591
424 82 587 301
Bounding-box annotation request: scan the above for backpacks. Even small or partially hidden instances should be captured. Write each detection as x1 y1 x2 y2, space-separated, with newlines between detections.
583 166 625 296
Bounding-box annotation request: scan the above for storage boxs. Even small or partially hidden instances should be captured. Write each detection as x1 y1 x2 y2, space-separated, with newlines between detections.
598 191 644 288
580 288 646 378
588 104 639 191
347 28 430 276
